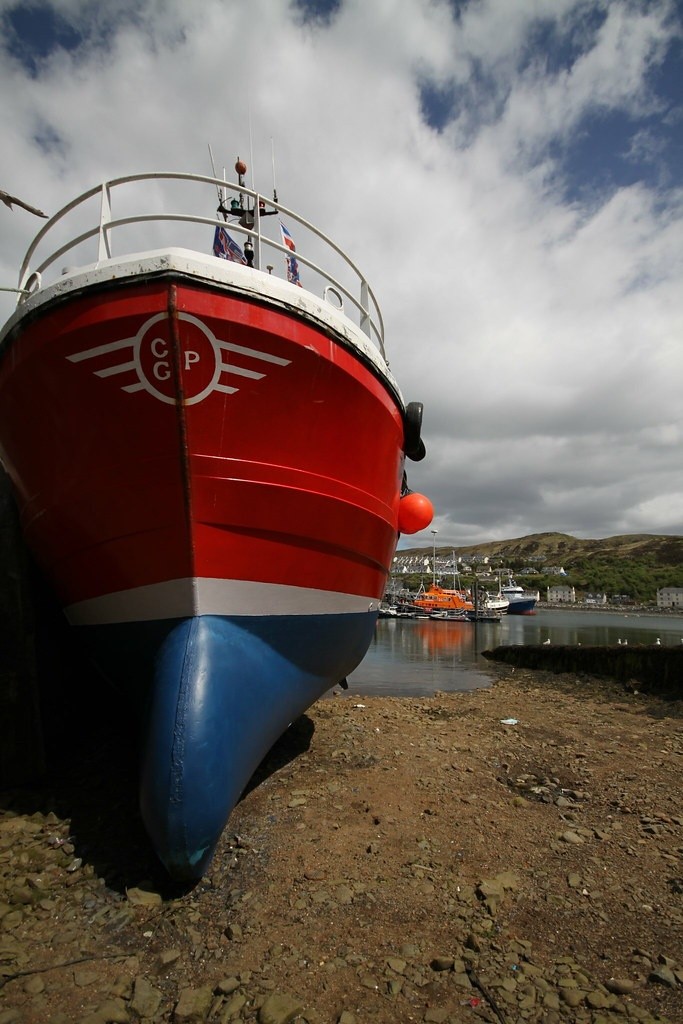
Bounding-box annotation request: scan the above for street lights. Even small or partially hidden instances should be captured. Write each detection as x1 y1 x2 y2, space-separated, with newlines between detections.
430 530 438 587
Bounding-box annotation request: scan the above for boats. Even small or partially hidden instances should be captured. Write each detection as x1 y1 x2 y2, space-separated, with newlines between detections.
380 574 538 624
1 164 436 910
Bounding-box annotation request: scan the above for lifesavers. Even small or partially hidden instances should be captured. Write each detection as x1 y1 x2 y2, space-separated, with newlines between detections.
405 401 426 462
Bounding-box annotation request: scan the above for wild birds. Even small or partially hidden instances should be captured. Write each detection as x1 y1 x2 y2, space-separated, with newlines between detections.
542 637 683 646
0 189 50 220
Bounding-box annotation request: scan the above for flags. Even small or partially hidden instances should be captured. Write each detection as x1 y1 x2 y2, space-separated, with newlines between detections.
280 222 303 287
213 226 247 265
